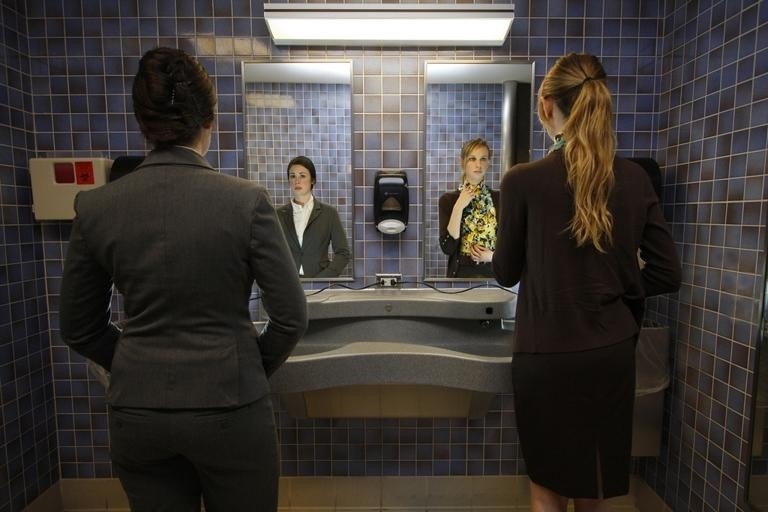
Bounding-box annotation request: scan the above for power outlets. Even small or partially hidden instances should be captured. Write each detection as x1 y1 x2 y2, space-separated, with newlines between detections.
380 276 400 288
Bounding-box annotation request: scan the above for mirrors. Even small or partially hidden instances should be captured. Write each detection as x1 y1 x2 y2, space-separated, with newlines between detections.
424 58 537 283
241 59 354 282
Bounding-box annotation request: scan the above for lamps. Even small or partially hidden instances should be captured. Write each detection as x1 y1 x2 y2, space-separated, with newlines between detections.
261 1 516 48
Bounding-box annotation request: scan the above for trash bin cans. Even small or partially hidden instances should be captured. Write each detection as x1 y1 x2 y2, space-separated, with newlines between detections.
624 318 670 458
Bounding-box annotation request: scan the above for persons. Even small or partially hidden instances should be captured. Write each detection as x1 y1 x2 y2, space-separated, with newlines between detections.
58 46 312 512
489 51 684 512
276 155 350 279
438 137 502 278
